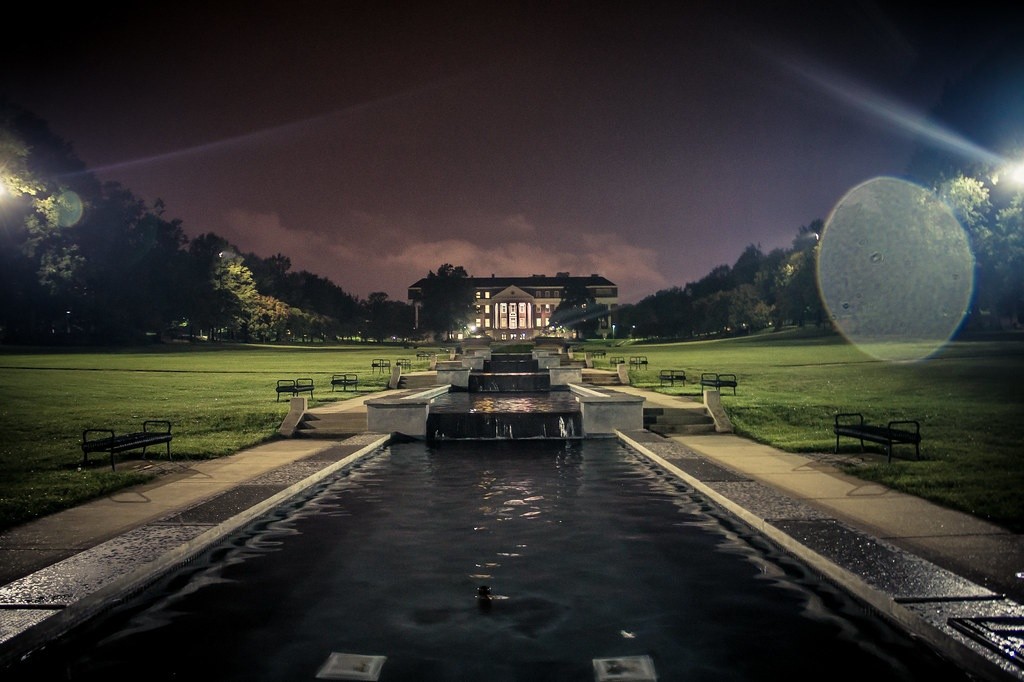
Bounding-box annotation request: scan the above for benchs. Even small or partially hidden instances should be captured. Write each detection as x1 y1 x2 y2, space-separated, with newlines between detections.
76 419 173 473
833 413 922 465
700 372 737 396
416 350 435 360
629 356 648 371
332 373 359 392
396 359 411 370
660 369 686 387
276 378 314 402
592 349 606 358
609 356 625 366
372 358 391 374
